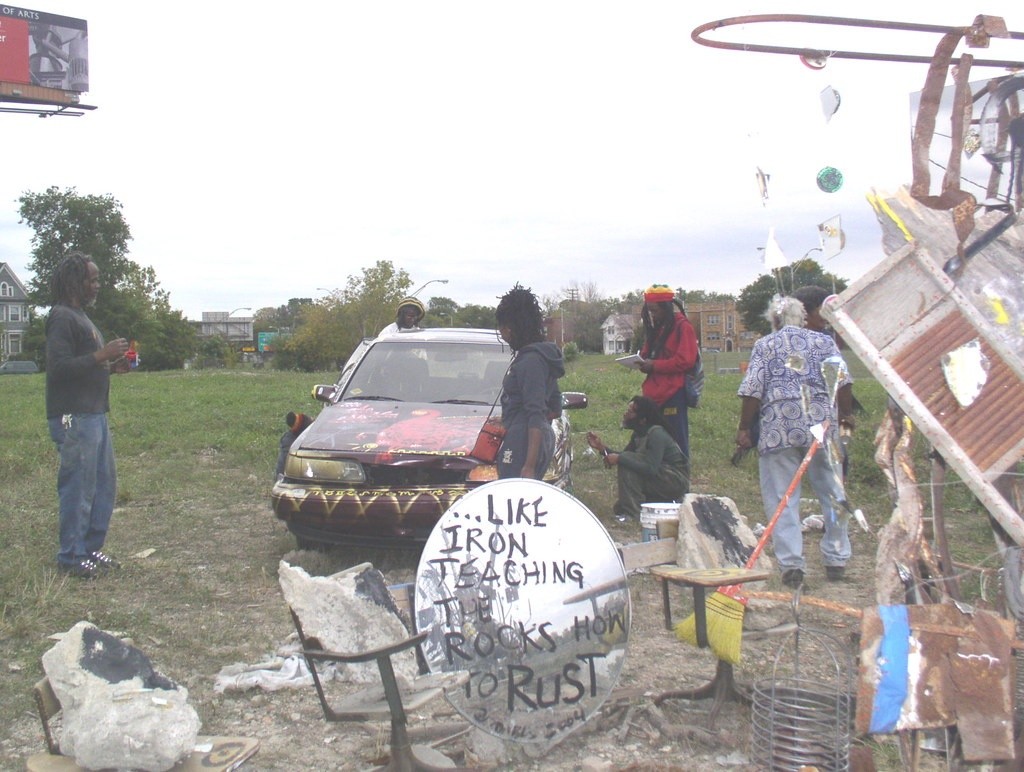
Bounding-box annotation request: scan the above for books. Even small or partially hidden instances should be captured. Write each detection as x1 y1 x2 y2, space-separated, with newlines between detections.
616 354 644 368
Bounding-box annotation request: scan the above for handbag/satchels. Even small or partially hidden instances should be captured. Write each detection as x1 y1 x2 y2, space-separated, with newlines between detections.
470 423 507 463
684 348 703 408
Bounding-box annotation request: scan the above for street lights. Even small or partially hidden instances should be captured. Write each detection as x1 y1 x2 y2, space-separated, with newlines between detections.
213 306 252 334
411 279 451 298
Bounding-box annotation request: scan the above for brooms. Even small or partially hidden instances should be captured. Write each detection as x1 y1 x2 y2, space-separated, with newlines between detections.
672 420 831 664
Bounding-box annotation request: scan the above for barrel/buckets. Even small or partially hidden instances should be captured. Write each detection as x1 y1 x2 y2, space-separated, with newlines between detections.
640 502 681 542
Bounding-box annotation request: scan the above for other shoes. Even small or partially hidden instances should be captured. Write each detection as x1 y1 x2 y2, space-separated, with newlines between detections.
782 569 802 588
826 565 845 581
615 514 638 524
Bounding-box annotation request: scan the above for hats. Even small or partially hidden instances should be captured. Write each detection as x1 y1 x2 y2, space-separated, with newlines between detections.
286 411 313 437
644 285 673 303
396 296 425 322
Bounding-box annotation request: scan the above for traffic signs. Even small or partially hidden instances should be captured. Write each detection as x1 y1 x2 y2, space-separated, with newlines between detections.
257 332 280 352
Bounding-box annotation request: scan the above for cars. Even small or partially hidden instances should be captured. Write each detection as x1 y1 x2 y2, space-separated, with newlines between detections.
269 328 588 553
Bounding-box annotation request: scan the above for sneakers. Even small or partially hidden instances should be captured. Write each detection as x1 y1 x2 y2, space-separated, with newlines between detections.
88 551 120 569
59 560 101 579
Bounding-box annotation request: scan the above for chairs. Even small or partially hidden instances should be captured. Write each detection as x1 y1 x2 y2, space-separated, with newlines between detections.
652 494 800 734
398 359 432 396
26 638 260 772
284 560 471 772
481 362 513 405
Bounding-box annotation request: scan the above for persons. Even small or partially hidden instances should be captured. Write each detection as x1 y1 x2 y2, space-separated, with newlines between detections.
795 287 835 340
275 411 313 482
495 280 563 480
736 297 854 584
378 296 428 360
43 251 140 576
586 396 688 524
634 284 699 469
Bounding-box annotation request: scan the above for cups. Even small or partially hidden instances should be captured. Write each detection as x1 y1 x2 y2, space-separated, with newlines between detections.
840 419 852 445
125 351 139 369
